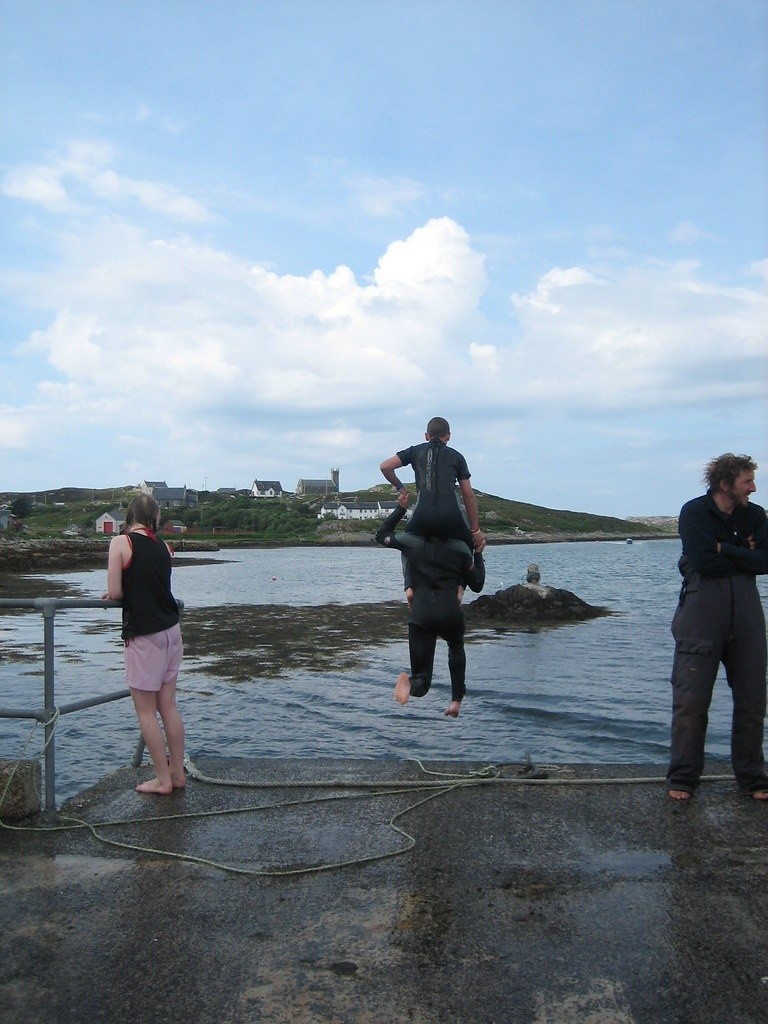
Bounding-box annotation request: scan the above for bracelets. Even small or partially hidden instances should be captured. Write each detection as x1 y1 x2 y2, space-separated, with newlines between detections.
393 483 403 490
472 530 480 534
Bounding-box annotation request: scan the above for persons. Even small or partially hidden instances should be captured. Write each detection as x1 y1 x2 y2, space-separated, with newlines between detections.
375 417 486 717
101 494 185 794
664 455 768 799
